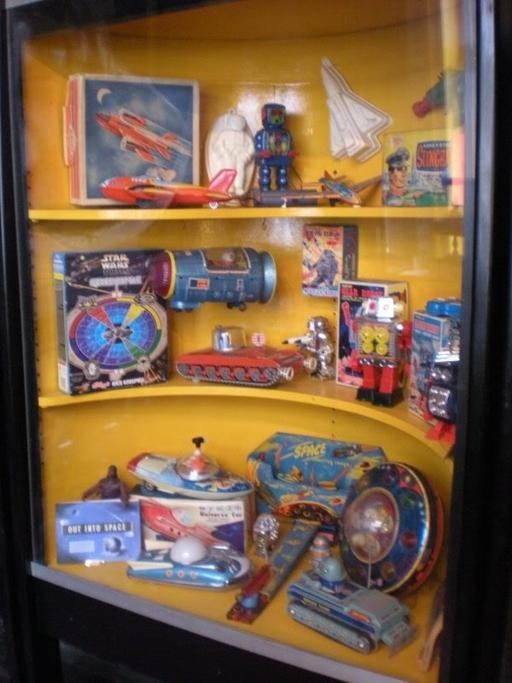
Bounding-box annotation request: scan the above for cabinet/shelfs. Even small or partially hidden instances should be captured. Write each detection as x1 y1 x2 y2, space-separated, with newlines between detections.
0 0 512 683
